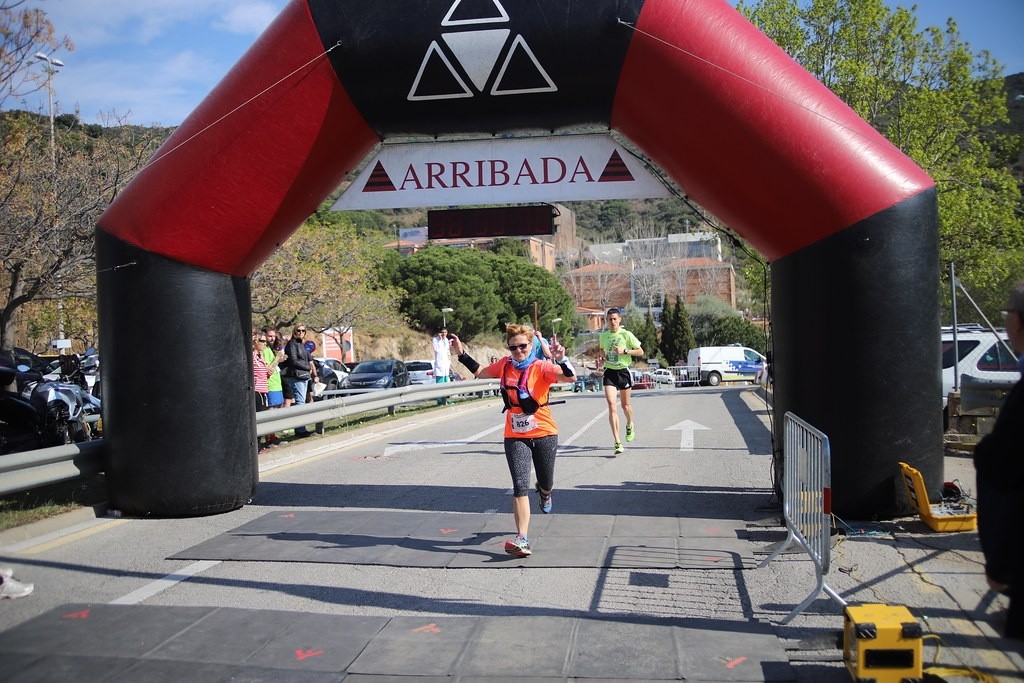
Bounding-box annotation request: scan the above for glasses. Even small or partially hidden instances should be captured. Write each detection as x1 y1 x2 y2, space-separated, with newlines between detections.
297 329 306 333
509 342 529 351
259 340 266 343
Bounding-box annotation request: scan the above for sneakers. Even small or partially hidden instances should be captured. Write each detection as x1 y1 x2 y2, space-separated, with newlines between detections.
258 448 268 455
535 483 552 513
614 442 624 453
504 535 532 557
626 423 634 442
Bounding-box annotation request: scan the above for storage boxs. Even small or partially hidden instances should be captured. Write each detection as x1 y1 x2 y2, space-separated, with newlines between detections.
899 460 977 532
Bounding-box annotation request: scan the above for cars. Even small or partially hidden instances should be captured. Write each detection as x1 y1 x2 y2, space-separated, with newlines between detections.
449 365 467 382
313 360 339 402
574 375 596 393
632 374 656 390
643 368 675 384
403 360 450 385
345 362 384 372
940 327 1022 432
339 359 412 397
314 358 351 382
0 353 100 456
675 374 694 388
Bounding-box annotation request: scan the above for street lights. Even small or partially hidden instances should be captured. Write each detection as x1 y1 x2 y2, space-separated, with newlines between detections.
551 318 562 364
33 52 65 356
442 308 454 327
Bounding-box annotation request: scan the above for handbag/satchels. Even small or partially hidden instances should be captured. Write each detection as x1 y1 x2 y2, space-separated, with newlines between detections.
312 378 327 397
295 369 310 380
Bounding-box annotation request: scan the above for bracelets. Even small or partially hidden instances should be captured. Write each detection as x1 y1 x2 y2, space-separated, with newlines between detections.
624 349 628 354
314 375 318 377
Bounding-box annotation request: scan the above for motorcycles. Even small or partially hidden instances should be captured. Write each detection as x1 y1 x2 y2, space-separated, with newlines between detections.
17 347 97 447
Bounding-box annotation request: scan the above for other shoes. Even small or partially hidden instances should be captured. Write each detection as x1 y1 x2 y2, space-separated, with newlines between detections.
293 431 309 438
271 437 280 444
267 443 278 448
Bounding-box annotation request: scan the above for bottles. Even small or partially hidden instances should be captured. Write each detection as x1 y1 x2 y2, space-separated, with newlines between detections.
520 390 528 399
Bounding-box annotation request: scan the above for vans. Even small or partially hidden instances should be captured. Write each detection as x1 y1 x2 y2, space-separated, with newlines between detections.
687 343 767 386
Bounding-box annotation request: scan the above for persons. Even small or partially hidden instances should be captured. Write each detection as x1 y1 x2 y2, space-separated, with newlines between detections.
972 281 1024 646
252 323 319 454
448 325 577 557
92 360 101 398
490 356 499 396
432 327 449 404
600 309 645 455
524 323 551 362
